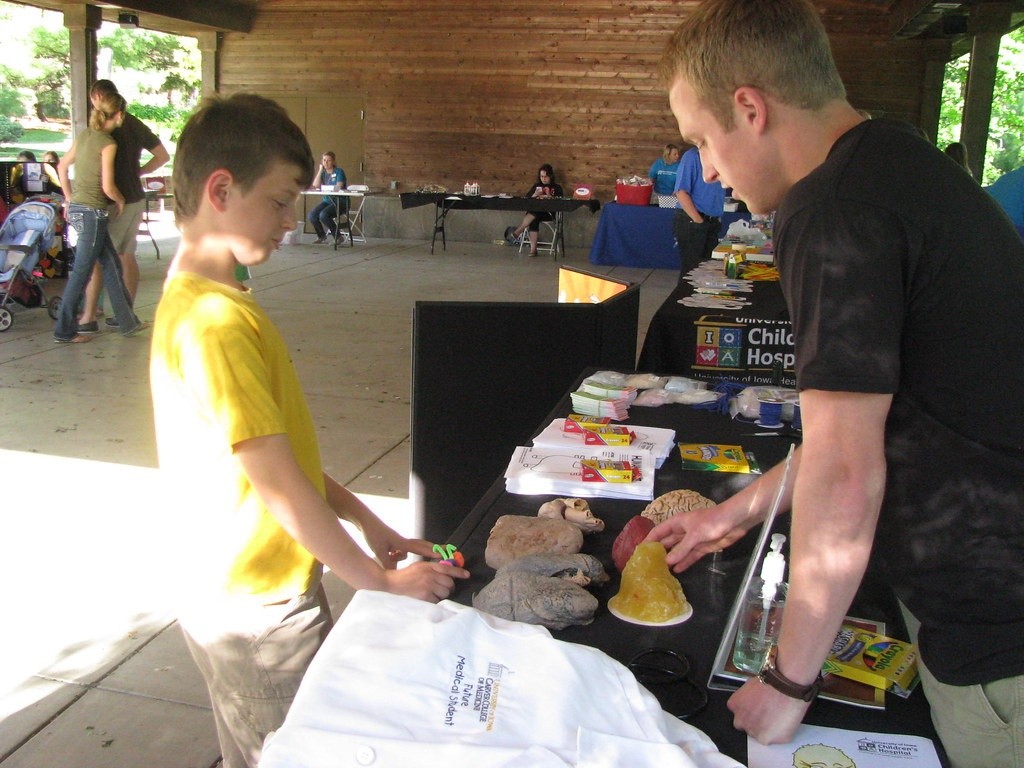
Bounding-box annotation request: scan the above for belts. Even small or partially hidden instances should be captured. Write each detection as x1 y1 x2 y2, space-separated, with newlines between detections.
697 211 722 223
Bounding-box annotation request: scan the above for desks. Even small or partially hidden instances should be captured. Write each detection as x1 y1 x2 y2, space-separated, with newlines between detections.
636 218 799 390
136 193 174 259
436 367 946 768
301 189 384 250
399 192 601 262
589 199 752 269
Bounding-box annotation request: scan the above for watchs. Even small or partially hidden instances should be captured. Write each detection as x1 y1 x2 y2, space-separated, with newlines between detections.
758 643 823 703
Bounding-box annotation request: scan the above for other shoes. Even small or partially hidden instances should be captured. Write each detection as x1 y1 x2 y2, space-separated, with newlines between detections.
528 249 538 257
105 315 142 328
124 322 149 336
312 235 327 244
511 232 519 239
334 236 344 245
75 322 102 332
54 332 92 344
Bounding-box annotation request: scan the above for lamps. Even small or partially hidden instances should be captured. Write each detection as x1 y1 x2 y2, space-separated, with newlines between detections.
117 9 139 29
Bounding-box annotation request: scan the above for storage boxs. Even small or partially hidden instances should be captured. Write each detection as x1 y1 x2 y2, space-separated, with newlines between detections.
678 439 750 474
726 254 744 279
817 624 922 699
564 414 632 484
615 183 654 207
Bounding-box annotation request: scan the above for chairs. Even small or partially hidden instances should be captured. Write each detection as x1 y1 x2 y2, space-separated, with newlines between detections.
518 208 561 255
325 185 369 244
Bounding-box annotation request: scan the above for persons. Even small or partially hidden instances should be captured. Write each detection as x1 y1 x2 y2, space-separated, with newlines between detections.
17 150 36 162
642 0 1024 767
648 144 681 196
675 147 725 284
42 150 60 162
147 93 470 768
854 107 1023 238
512 164 563 257
53 93 150 344
76 78 170 333
308 151 351 245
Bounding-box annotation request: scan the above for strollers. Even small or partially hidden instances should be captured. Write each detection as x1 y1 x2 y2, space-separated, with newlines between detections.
0 193 66 333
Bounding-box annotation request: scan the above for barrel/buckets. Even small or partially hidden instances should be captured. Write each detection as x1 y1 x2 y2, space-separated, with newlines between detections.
615 177 655 205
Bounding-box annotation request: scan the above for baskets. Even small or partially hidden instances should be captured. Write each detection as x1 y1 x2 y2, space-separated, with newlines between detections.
658 195 678 209
